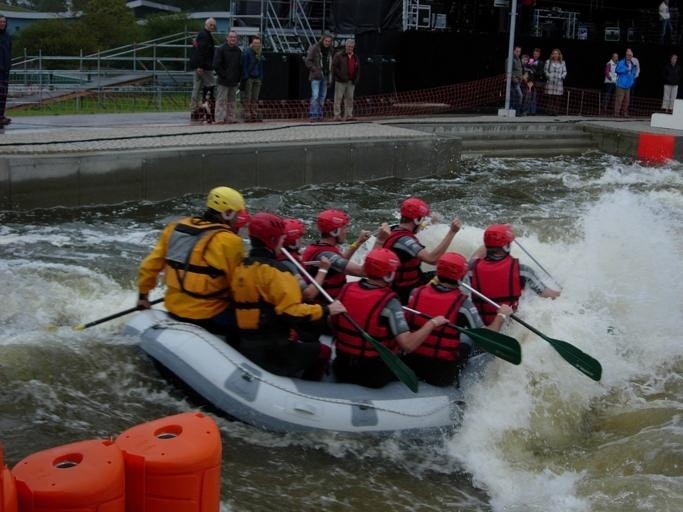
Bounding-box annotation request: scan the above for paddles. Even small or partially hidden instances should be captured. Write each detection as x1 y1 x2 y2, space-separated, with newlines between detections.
280 244 418 393
461 281 602 381
401 304 522 366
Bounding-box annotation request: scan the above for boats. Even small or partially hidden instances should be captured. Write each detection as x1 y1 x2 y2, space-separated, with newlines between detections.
117 308 518 453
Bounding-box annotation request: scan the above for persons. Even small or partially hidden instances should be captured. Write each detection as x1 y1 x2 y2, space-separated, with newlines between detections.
301 207 389 330
231 212 346 381
468 224 560 327
188 18 215 118
330 247 448 388
401 252 512 386
304 34 334 122
506 45 680 116
332 38 360 122
135 186 246 331
213 30 242 122
0 16 12 127
242 36 263 124
382 198 460 310
274 219 330 302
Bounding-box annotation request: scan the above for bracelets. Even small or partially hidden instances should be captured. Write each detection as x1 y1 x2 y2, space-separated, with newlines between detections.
351 242 360 249
317 269 327 275
374 241 384 246
420 222 428 228
430 318 443 331
429 278 440 284
498 312 507 319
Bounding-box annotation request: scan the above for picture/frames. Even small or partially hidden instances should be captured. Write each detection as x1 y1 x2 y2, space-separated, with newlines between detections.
431 13 447 28
604 26 620 41
627 27 641 42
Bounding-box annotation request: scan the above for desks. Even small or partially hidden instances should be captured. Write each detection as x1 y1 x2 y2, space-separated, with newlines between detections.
533 9 581 40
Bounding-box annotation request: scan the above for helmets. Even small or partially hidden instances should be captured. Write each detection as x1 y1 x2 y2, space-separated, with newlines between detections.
484 224 515 248
206 186 349 248
401 197 429 219
437 252 468 280
364 248 400 277
